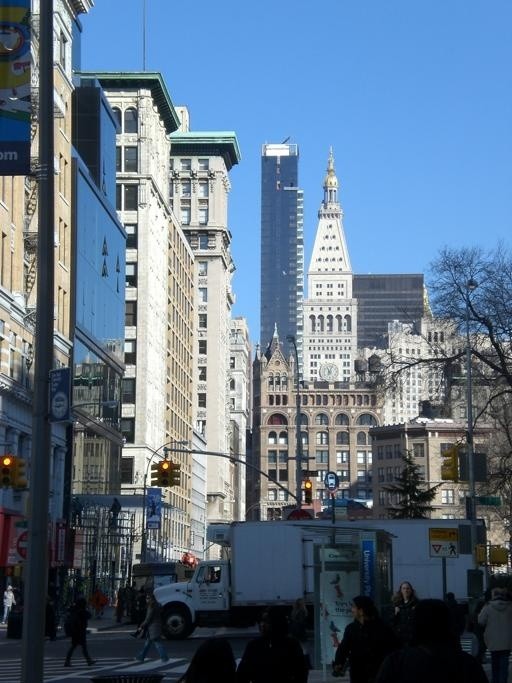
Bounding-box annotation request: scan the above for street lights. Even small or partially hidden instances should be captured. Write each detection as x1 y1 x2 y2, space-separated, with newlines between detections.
448 279 477 520
62 400 118 567
141 439 188 565
286 335 302 510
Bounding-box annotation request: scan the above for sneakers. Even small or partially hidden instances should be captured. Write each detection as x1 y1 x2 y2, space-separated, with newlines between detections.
2 621 5 625
88 661 96 666
134 656 145 662
129 633 137 638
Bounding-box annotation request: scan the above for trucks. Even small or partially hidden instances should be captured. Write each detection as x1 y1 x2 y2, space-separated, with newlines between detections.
153 517 488 642
131 563 198 626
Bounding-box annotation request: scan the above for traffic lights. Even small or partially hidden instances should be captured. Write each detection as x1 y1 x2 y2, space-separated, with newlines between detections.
304 480 313 504
150 458 179 487
471 545 486 564
14 458 29 489
1 455 16 489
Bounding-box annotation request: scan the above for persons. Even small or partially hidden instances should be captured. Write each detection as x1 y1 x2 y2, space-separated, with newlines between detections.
129 588 169 661
62 598 97 666
175 599 313 682
204 570 216 583
72 496 84 527
115 581 145 624
108 496 122 529
331 573 511 682
89 584 108 619
47 583 59 642
3 584 17 626
